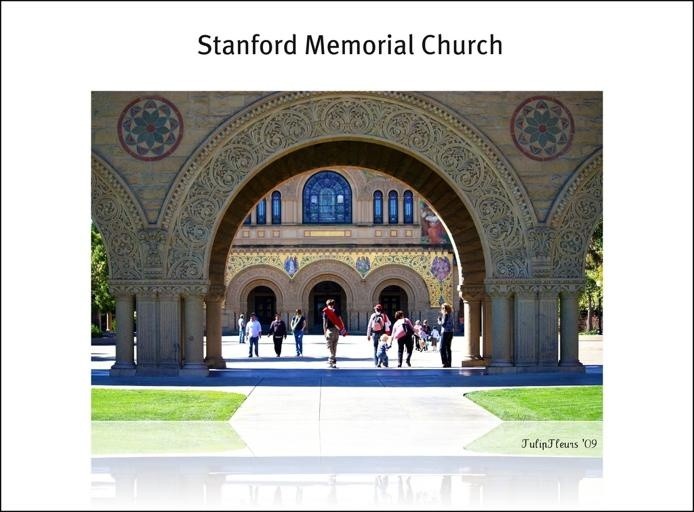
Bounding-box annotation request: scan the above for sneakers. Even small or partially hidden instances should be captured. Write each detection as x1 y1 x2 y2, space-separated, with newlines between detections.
329 362 336 367
248 354 260 358
397 361 411 368
377 365 389 368
296 354 303 357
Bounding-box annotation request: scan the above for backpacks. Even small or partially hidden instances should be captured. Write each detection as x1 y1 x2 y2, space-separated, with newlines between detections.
372 312 383 332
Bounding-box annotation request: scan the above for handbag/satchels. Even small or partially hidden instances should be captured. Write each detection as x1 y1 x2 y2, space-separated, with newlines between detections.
384 312 390 334
402 322 415 335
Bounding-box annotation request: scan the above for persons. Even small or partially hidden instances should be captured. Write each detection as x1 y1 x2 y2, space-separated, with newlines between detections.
237 314 246 343
244 312 262 358
322 299 346 368
367 303 455 368
291 309 307 358
268 314 287 357
